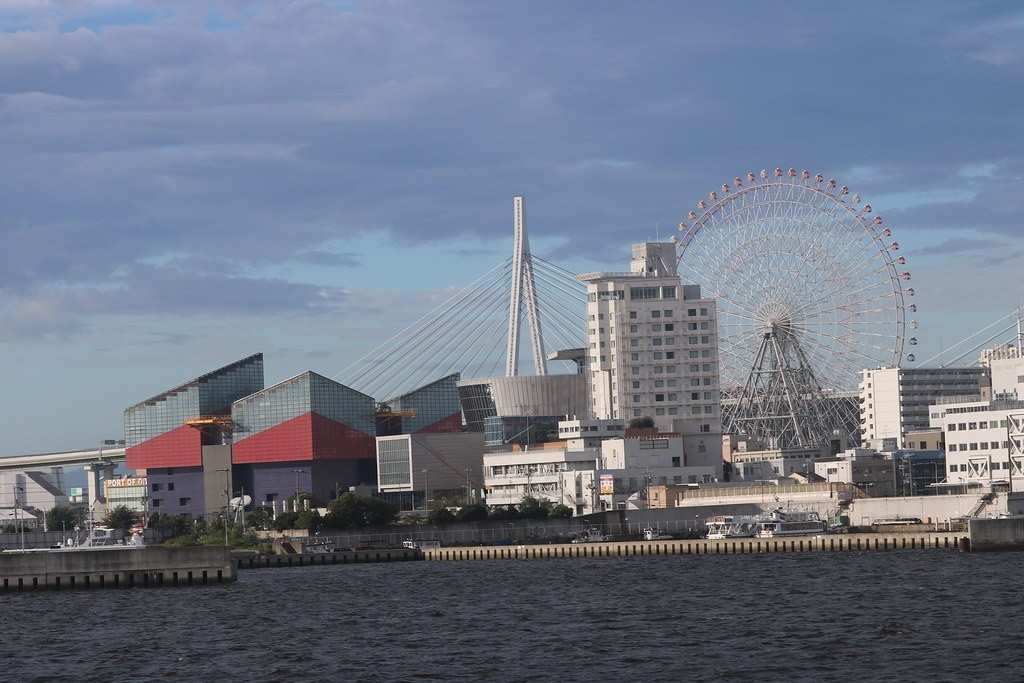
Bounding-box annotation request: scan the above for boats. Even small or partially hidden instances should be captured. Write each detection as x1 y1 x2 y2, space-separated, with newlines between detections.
644 527 674 541
570 528 612 544
705 505 825 540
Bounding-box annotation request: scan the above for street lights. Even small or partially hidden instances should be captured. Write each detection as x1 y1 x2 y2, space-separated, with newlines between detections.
858 482 875 498
